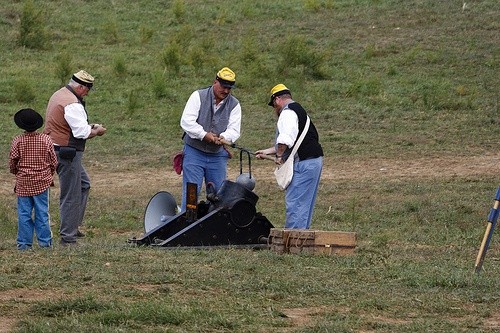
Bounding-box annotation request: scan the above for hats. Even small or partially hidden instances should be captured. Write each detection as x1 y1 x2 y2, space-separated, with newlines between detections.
72 70 95 88
14 108 44 132
215 67 238 88
268 83 291 106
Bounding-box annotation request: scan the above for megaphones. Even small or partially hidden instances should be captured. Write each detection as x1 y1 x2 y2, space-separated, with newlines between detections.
144 190 183 234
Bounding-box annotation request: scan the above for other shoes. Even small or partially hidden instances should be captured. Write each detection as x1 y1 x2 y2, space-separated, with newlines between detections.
74 231 87 239
59 240 81 248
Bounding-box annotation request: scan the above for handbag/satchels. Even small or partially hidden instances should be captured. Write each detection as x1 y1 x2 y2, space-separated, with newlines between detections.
274 155 294 192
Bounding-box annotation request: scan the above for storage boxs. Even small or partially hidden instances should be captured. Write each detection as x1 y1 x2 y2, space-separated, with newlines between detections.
270 228 356 257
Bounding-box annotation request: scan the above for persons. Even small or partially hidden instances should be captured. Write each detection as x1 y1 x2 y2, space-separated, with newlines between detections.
173 67 241 213
9 108 58 250
255 84 324 230
43 71 106 247
